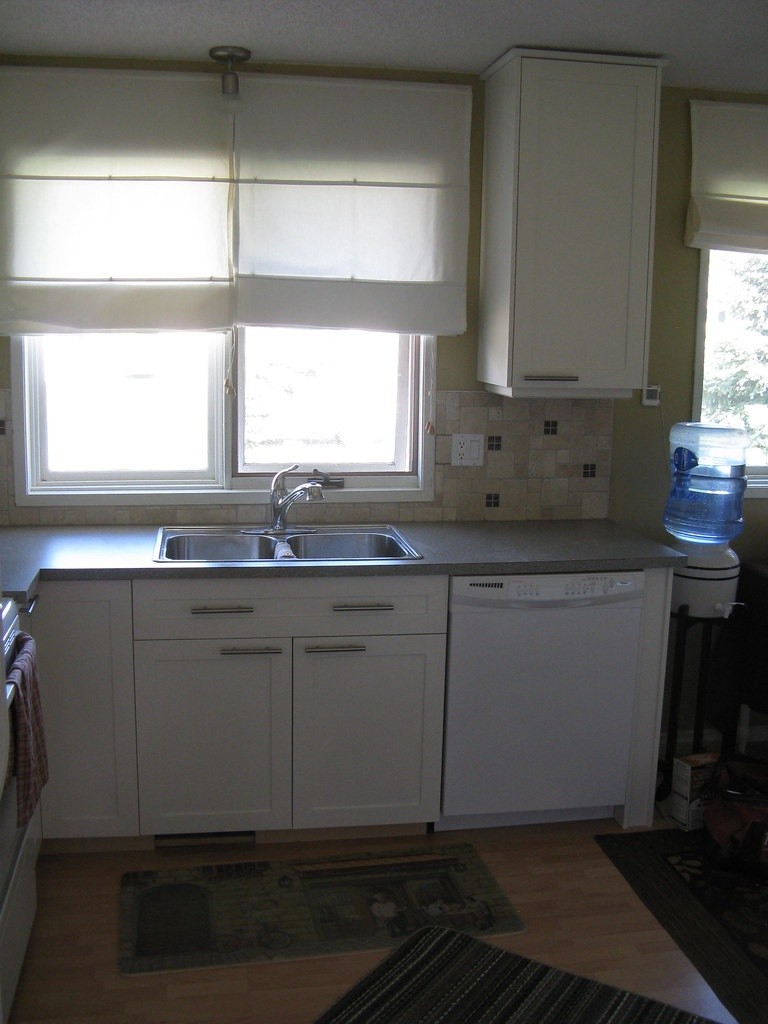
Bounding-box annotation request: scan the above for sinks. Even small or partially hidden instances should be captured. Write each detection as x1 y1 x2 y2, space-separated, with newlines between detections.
154 534 285 563
286 533 421 562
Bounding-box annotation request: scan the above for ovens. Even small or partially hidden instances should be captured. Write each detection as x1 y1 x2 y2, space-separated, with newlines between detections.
0 617 43 1024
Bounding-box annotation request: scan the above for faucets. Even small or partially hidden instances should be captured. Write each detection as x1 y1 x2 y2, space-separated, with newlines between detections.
714 602 745 619
270 464 326 530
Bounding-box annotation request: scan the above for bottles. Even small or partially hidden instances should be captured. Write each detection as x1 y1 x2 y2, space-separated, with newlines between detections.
662 421 747 545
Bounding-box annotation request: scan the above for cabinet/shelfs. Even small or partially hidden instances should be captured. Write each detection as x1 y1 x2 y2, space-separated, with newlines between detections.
473 47 659 401
23 575 451 844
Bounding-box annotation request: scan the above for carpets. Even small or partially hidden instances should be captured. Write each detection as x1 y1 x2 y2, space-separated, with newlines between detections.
596 828 768 1024
311 927 716 1024
115 841 527 978
